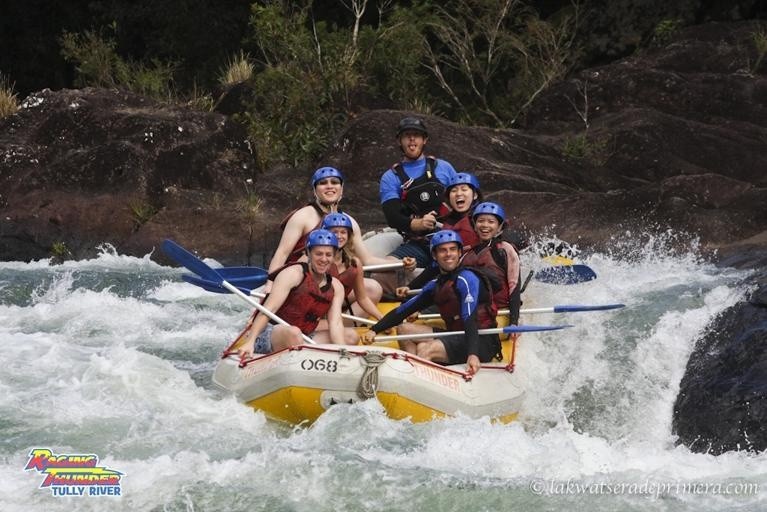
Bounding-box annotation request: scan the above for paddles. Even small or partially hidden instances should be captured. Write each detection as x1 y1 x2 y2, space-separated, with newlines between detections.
416 304 625 319
180 275 378 326
433 221 575 265
373 324 575 342
164 238 317 345
201 257 412 289
398 264 597 294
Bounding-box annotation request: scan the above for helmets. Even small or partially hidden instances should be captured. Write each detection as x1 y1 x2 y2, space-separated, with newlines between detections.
445 173 480 191
321 213 351 230
310 166 343 190
395 117 427 139
471 202 504 221
305 229 338 250
429 230 462 255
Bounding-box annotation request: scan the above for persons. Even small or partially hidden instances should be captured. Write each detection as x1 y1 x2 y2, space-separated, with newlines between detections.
361 230 503 374
239 229 359 358
295 213 392 331
379 117 457 279
265 166 417 327
395 172 483 298
395 202 521 341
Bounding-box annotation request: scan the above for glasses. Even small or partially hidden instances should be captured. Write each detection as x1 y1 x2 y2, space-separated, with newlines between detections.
319 178 340 185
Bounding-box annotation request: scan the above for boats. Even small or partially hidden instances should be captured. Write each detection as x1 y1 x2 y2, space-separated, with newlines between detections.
208 227 528 433
